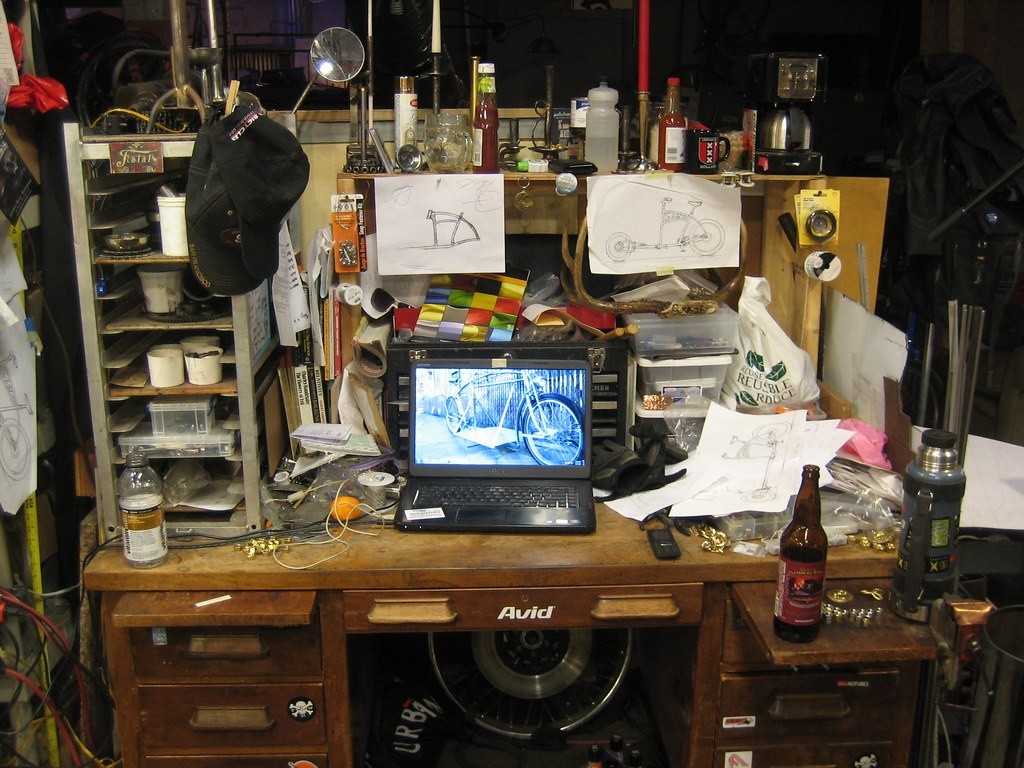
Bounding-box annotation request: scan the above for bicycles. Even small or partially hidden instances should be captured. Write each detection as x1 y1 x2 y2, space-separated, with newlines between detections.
443 369 584 465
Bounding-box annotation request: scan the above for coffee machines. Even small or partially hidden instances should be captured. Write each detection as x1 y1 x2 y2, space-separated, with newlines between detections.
744 52 822 175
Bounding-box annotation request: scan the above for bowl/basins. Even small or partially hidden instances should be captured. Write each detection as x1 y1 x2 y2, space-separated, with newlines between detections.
105 233 150 250
148 335 222 387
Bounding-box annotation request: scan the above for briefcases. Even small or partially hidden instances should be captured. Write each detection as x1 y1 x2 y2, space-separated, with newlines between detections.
720 275 819 414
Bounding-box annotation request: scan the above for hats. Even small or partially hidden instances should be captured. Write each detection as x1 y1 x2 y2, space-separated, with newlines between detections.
185 101 310 296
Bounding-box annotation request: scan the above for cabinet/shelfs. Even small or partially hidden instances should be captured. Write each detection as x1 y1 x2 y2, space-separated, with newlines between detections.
64 110 302 538
84 503 938 768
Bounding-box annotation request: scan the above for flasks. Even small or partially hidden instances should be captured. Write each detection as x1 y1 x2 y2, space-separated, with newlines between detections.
886 428 966 621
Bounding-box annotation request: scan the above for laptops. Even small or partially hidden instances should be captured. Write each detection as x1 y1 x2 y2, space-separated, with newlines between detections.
394 360 596 534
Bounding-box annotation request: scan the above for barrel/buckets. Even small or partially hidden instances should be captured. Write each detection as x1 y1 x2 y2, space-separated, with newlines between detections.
157 197 189 257
137 265 183 313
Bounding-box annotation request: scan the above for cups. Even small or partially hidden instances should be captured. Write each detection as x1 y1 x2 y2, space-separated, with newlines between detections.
686 130 730 174
423 113 472 173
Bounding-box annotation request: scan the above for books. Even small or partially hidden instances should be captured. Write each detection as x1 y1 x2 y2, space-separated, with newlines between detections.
278 285 341 460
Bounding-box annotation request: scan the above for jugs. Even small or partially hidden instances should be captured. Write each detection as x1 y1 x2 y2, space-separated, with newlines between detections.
758 101 812 151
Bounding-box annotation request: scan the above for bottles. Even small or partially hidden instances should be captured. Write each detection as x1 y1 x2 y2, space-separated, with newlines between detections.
774 462 828 643
656 78 686 169
118 452 168 568
584 82 619 172
472 63 500 174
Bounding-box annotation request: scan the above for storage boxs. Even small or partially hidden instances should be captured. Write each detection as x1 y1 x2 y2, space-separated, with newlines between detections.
615 300 744 417
118 420 236 459
146 396 217 436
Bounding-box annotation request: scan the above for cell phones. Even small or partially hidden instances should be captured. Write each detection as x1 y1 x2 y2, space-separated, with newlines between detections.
648 529 681 560
